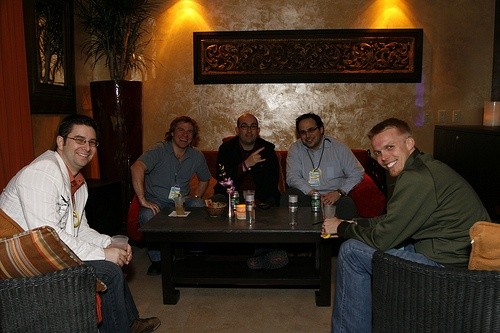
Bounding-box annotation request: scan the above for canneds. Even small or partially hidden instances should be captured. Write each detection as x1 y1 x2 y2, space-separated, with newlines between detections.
310 192 321 211
231 191 239 212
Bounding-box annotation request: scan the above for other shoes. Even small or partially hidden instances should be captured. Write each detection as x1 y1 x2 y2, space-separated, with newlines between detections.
132 315 161 333
148 261 162 275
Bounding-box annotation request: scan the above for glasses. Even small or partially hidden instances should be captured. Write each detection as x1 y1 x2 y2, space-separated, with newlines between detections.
239 125 258 130
299 127 318 134
64 136 99 147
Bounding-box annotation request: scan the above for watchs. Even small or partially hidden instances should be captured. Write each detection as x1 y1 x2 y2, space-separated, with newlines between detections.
337 189 344 197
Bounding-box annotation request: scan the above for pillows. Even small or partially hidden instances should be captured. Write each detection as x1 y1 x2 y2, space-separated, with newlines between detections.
1 209 24 239
468 221 500 270
0 226 107 292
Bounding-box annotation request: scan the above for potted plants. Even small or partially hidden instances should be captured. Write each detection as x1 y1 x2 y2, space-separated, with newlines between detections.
73 0 157 183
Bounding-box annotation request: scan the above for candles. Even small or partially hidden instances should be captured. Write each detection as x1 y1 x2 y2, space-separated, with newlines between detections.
483 101 500 126
452 110 463 123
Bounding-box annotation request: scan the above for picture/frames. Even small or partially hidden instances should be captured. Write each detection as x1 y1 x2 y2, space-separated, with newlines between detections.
21 0 75 115
192 29 422 85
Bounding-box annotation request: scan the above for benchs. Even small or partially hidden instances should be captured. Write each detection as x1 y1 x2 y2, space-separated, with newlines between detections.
126 151 369 240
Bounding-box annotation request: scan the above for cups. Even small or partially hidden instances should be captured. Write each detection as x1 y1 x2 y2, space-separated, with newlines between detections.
321 203 330 219
174 197 185 215
324 205 336 220
243 190 255 204
483 102 500 126
110 235 129 252
288 195 298 213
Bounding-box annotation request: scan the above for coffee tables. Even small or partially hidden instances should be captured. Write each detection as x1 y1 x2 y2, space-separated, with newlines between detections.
140 204 343 307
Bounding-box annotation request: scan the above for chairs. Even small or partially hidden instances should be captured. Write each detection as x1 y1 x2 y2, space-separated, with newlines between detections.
0 263 100 333
371 252 500 333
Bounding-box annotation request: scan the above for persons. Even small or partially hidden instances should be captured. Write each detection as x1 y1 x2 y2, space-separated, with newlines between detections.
282 112 365 219
131 116 211 255
321 117 493 333
0 113 161 333
213 113 280 208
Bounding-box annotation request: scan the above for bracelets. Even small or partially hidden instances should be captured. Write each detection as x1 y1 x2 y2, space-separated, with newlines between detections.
193 193 199 198
243 161 250 171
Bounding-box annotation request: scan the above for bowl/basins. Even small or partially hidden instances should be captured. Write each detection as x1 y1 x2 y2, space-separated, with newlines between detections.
204 204 228 217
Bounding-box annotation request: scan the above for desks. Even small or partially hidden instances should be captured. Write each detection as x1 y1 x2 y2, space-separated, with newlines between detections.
85 180 126 235
434 124 500 224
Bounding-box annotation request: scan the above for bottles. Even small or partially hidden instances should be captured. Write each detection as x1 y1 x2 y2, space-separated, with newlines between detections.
232 191 255 220
311 191 321 212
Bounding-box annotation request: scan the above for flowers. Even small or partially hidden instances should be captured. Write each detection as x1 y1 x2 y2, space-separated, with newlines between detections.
218 163 236 193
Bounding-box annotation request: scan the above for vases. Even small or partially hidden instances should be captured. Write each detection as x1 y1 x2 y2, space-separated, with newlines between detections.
226 190 234 218
